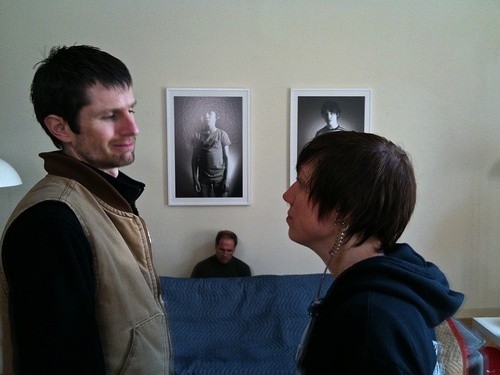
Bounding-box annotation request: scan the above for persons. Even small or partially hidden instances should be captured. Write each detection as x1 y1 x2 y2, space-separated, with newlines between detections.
191 107 232 198
282 131 464 375
0 45 172 375
191 231 251 278
315 99 346 135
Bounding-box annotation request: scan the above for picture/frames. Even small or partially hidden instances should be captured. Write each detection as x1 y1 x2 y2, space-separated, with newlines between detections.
288 87 372 188
165 87 252 207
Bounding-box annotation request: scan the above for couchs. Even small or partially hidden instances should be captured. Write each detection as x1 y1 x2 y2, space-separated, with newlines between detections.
158 273 483 375
